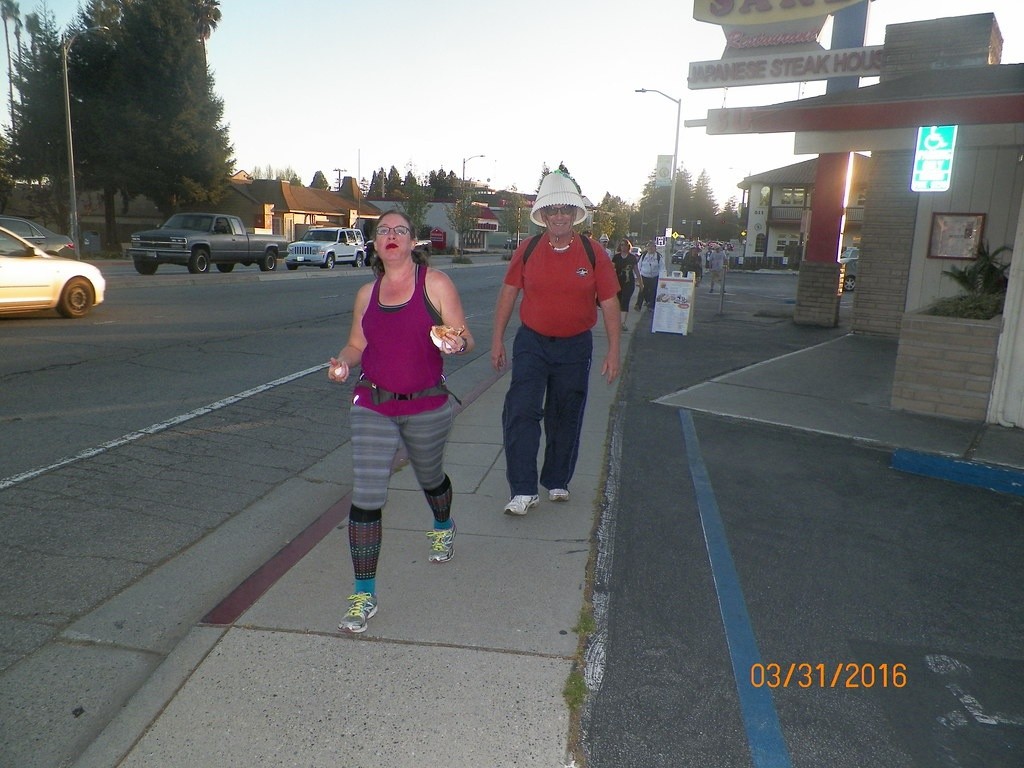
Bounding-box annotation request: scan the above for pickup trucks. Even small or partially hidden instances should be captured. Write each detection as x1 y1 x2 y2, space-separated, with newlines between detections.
126 212 280 275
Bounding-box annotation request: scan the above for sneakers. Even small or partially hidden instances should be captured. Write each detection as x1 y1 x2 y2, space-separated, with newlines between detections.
548 489 570 502
426 517 457 564
337 591 378 634
504 494 539 516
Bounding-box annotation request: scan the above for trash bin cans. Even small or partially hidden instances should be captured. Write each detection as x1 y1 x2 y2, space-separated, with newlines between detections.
82 230 101 251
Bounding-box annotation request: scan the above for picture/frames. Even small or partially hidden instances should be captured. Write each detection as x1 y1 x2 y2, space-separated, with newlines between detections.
926 211 986 261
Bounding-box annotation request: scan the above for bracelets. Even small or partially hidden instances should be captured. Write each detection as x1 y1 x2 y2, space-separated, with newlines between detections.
457 337 468 351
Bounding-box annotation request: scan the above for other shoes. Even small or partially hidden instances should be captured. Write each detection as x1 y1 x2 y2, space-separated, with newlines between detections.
621 322 628 331
634 305 641 312
709 289 714 293
597 305 602 310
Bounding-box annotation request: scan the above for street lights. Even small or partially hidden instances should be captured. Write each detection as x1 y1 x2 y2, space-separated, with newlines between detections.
62 25 109 261
635 88 682 277
463 155 485 181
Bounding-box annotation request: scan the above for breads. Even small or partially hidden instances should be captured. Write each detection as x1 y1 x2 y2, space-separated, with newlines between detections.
435 324 465 341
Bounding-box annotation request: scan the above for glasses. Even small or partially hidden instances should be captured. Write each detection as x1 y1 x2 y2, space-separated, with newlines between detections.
375 225 414 241
544 205 574 216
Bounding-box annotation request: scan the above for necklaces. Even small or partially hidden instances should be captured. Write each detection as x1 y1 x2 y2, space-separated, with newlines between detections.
549 231 575 251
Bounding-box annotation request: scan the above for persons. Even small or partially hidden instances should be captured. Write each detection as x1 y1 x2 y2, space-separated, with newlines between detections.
634 242 664 313
329 209 472 633
709 246 726 293
493 174 621 515
582 230 592 237
596 233 611 309
612 239 644 331
680 247 703 287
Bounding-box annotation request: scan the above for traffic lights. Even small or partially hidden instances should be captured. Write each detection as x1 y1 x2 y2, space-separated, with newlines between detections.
741 231 746 236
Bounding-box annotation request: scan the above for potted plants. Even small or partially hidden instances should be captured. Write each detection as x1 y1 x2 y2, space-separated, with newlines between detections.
889 235 1012 420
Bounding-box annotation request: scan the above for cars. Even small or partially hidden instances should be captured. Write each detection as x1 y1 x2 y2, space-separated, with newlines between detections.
674 239 735 252
671 249 685 265
840 245 861 291
504 237 523 250
0 215 77 260
630 247 643 258
0 226 108 320
363 240 378 267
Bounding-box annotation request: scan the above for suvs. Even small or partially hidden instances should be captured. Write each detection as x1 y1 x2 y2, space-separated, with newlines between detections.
283 226 367 271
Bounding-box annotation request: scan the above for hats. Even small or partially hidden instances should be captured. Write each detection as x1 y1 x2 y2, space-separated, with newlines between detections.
530 170 588 228
599 234 609 242
